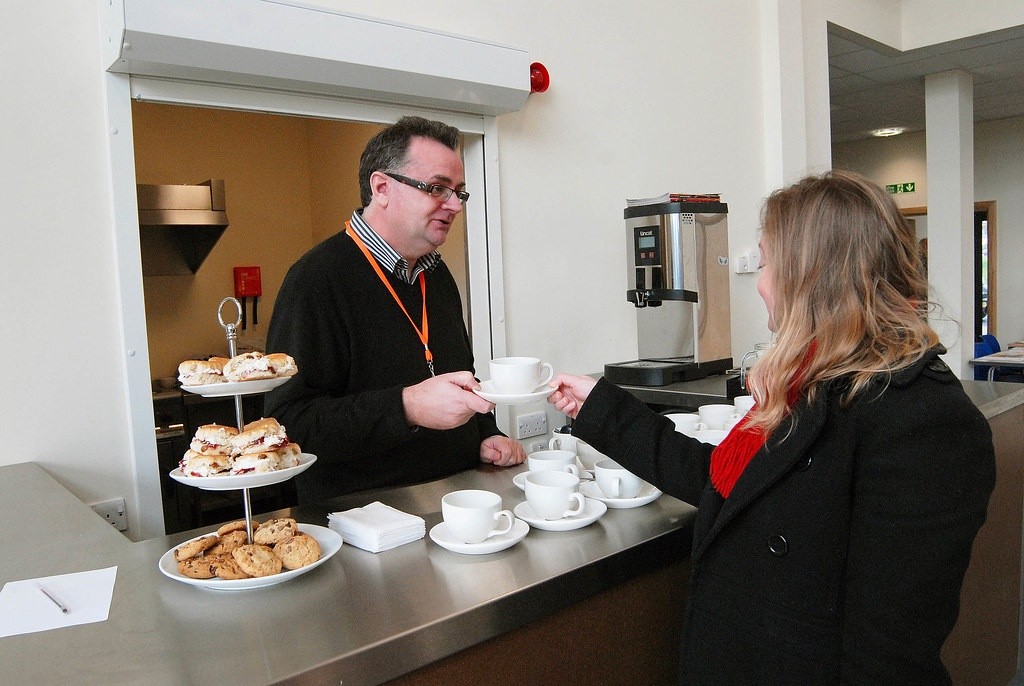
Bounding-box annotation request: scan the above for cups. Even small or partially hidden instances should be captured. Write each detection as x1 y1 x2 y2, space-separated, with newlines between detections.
741 342 769 389
161 378 176 388
664 395 756 446
488 356 553 395
441 490 515 544
525 427 644 520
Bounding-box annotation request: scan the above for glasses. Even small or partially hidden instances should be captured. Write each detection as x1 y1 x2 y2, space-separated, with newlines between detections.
369 169 469 205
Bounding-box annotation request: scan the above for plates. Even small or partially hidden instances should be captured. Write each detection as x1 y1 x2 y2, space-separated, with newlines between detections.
512 470 593 492
180 376 292 398
169 453 318 491
514 497 607 531
429 517 530 555
158 523 343 589
580 480 663 508
473 381 560 406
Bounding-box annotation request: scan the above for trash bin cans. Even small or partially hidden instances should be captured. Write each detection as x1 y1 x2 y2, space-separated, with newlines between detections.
974 335 1002 380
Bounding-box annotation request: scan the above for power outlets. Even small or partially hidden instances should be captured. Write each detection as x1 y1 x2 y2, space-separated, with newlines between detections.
517 409 549 442
89 499 128 535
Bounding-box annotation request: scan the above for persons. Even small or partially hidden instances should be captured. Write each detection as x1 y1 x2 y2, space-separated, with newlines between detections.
546 167 996 686
263 115 526 505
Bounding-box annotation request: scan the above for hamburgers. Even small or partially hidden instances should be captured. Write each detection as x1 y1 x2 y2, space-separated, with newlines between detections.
179 418 301 478
178 350 298 386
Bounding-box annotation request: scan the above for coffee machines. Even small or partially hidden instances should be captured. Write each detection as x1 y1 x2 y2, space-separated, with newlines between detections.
604 202 733 387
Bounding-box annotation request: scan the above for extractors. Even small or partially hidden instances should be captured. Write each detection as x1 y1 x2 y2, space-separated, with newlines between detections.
137 179 230 277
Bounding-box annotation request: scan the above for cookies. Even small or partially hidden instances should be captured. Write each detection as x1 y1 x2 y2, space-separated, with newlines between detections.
174 517 320 580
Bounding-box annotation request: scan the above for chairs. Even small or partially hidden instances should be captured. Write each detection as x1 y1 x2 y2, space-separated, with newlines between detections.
973 334 1024 382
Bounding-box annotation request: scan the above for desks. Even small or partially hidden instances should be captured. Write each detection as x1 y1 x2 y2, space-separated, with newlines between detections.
967 344 1024 382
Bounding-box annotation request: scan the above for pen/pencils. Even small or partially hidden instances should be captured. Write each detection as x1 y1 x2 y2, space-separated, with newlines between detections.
40 589 69 615
